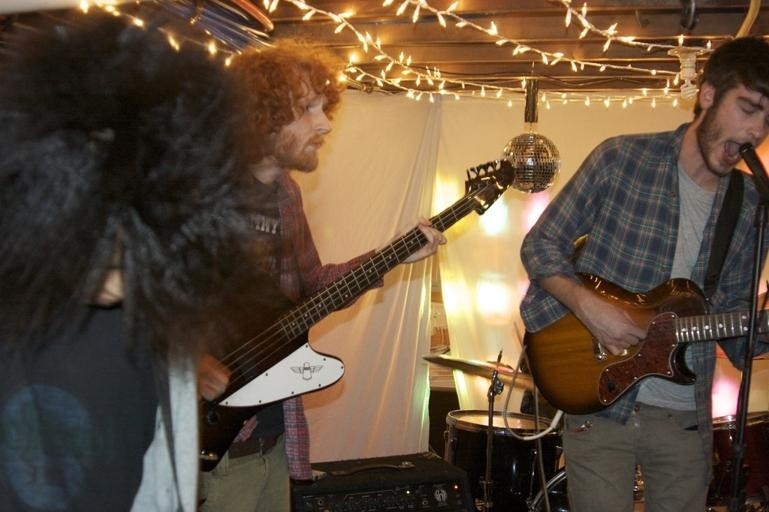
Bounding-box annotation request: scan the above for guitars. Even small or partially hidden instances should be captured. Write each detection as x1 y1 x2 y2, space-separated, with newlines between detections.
522 272 769 416
200 160 516 473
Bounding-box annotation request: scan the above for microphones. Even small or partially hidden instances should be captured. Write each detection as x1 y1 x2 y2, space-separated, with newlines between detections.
739 142 769 204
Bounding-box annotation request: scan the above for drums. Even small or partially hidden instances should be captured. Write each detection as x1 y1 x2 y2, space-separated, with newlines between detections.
444 409 562 505
712 410 769 504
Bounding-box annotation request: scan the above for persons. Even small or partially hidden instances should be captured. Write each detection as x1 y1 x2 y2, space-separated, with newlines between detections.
193 49 448 508
0 8 261 509
516 34 767 511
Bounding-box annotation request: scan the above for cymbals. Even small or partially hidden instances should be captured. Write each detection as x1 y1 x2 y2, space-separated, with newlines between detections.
421 355 534 391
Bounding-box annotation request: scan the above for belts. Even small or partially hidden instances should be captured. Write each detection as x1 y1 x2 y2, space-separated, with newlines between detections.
228 428 277 457
